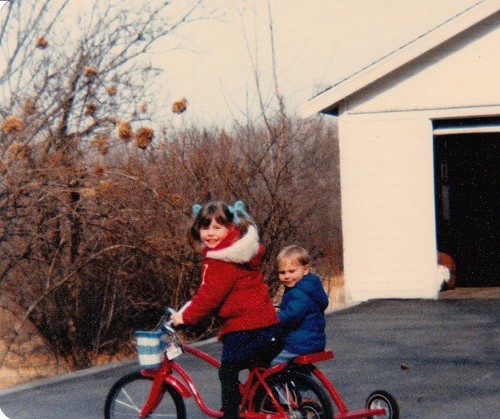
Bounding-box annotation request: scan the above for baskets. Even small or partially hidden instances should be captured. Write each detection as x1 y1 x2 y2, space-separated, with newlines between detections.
134 328 168 370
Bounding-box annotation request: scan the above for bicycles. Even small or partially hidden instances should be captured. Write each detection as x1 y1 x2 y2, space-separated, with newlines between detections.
103 299 335 419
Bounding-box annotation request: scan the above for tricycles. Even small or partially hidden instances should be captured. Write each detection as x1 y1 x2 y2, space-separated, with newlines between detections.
272 303 401 419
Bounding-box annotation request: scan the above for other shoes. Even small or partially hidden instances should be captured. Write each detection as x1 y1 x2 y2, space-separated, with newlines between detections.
263 402 291 414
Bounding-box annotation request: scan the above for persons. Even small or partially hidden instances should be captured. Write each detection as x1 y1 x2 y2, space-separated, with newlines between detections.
270 244 329 394
170 200 281 419
